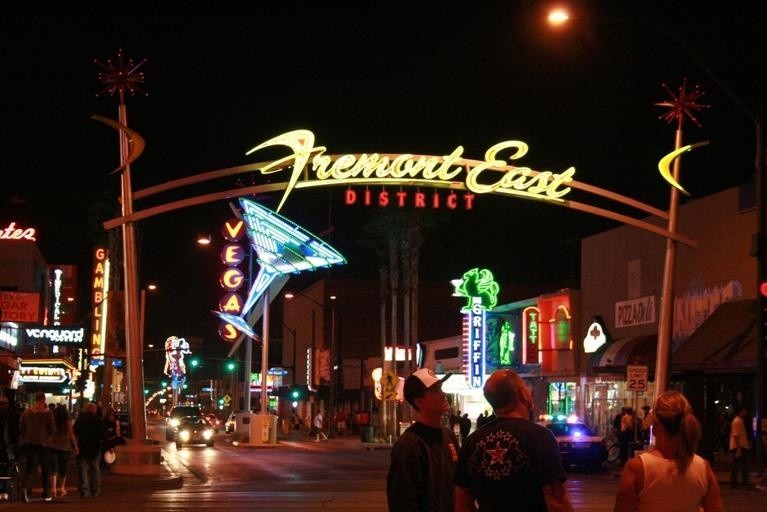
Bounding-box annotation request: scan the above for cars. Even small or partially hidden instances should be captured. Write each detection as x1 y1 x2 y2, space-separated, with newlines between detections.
532 421 612 474
147 407 161 415
163 406 236 450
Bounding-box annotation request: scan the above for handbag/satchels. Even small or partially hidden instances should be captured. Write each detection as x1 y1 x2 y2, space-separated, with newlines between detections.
104 450 116 464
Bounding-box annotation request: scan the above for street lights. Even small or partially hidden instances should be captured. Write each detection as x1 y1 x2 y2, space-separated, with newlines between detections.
133 209 211 292
68 281 157 414
282 288 336 441
141 343 155 350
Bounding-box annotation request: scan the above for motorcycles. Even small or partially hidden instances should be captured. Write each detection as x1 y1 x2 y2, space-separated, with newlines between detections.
601 425 649 462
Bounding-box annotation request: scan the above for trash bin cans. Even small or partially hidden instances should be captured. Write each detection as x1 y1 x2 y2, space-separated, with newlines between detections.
359 425 375 442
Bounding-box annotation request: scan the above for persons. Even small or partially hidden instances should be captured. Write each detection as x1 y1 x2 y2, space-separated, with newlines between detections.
612 404 656 467
452 368 575 512
384 374 393 387
754 411 767 492
612 390 726 512
0 389 116 504
291 407 369 442
726 405 755 490
385 366 478 511
171 339 188 372
448 407 496 448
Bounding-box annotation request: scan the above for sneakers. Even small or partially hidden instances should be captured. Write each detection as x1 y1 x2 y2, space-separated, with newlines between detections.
41 495 52 501
23 496 29 502
80 490 89 497
59 487 67 494
51 488 57 499
93 488 101 496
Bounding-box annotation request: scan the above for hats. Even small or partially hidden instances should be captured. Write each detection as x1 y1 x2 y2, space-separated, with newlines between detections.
404 367 453 402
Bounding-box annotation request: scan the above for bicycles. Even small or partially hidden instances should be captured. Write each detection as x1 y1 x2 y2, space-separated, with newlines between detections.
1 443 25 501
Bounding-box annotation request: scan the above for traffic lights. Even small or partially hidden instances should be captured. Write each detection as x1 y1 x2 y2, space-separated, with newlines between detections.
290 390 298 408
217 398 225 411
